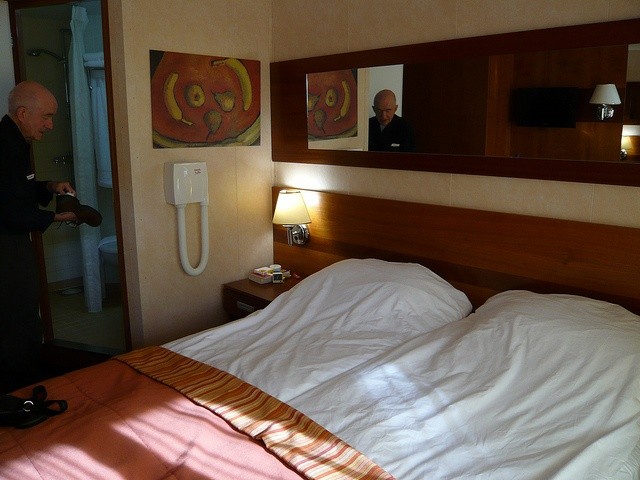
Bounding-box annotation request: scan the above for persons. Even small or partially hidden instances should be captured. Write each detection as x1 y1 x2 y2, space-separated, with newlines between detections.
0 81 102 396
368 89 410 151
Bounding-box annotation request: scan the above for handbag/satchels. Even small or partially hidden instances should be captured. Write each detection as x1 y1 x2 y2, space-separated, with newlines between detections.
0 385 68 429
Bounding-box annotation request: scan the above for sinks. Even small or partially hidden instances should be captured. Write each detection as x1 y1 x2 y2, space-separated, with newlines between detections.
99 243 120 269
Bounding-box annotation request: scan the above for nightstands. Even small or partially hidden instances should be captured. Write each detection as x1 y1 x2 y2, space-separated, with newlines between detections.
223 277 303 321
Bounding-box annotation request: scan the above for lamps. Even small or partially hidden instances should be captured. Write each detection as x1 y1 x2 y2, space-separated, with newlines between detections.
589 84 620 124
272 189 311 248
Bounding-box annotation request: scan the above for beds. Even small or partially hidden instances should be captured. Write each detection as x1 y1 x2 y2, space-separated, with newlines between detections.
1 258 640 480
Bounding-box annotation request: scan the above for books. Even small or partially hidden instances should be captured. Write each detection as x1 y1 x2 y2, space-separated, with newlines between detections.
249 268 291 284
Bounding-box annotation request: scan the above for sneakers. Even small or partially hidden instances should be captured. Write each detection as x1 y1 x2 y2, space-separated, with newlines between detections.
56 195 102 231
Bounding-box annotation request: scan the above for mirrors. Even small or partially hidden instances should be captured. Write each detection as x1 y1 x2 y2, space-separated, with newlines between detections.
305 65 403 152
